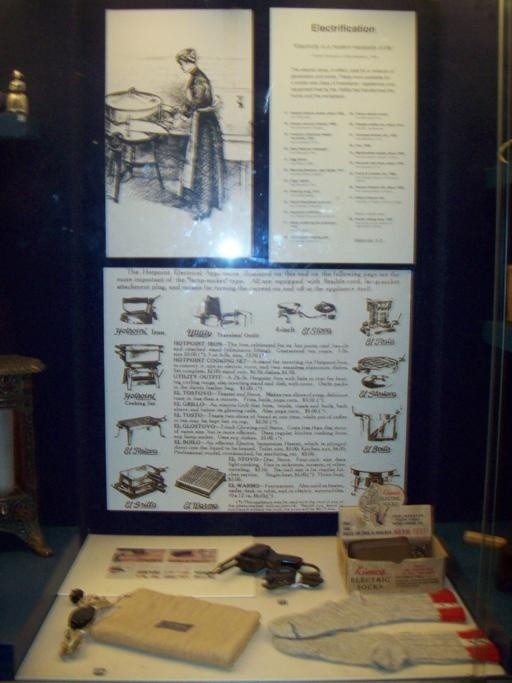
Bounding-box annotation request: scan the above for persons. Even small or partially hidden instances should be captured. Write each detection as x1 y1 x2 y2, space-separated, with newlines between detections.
172 47 231 220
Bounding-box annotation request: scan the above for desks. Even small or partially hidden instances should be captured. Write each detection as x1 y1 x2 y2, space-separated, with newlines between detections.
105 121 166 204
